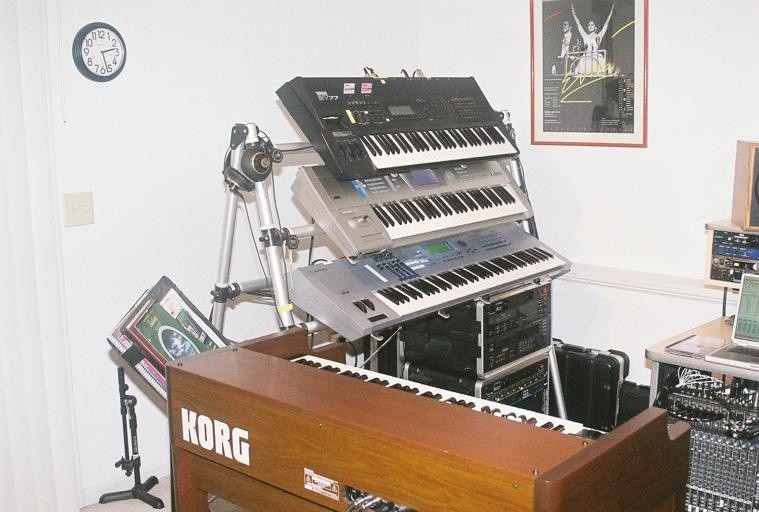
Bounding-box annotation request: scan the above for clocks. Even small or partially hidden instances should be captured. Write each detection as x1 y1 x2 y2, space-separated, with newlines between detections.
72 22 127 83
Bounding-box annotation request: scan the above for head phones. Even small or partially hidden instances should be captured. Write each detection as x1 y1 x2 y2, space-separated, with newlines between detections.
256 131 283 163
222 136 272 193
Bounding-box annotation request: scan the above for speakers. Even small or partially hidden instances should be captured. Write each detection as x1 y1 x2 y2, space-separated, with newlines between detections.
731 140 759 231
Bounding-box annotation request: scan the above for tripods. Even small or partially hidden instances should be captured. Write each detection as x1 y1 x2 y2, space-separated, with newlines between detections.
99 367 163 509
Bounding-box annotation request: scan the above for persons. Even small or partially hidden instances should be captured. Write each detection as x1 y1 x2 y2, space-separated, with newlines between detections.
570 1 617 76
551 19 584 75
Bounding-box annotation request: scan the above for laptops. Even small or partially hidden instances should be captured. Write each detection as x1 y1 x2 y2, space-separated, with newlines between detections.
705 273 759 371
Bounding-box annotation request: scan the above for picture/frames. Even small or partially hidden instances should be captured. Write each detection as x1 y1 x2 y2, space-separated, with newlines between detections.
530 0 649 149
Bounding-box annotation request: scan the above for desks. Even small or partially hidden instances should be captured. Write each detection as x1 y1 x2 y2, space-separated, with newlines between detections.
644 218 758 409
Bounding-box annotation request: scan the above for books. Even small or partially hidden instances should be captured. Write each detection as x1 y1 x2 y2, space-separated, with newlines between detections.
105 275 232 402
